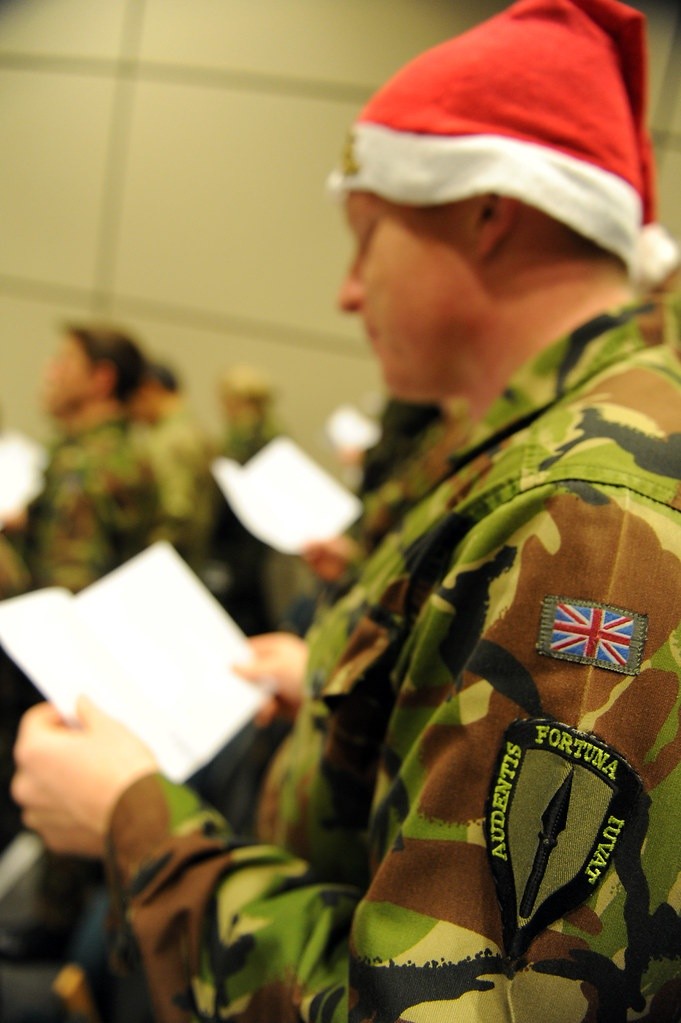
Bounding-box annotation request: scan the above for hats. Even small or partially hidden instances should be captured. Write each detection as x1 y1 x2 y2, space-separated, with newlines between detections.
328 0 681 283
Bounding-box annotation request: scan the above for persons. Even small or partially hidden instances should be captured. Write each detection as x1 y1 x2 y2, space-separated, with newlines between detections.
0 0 681 1023
